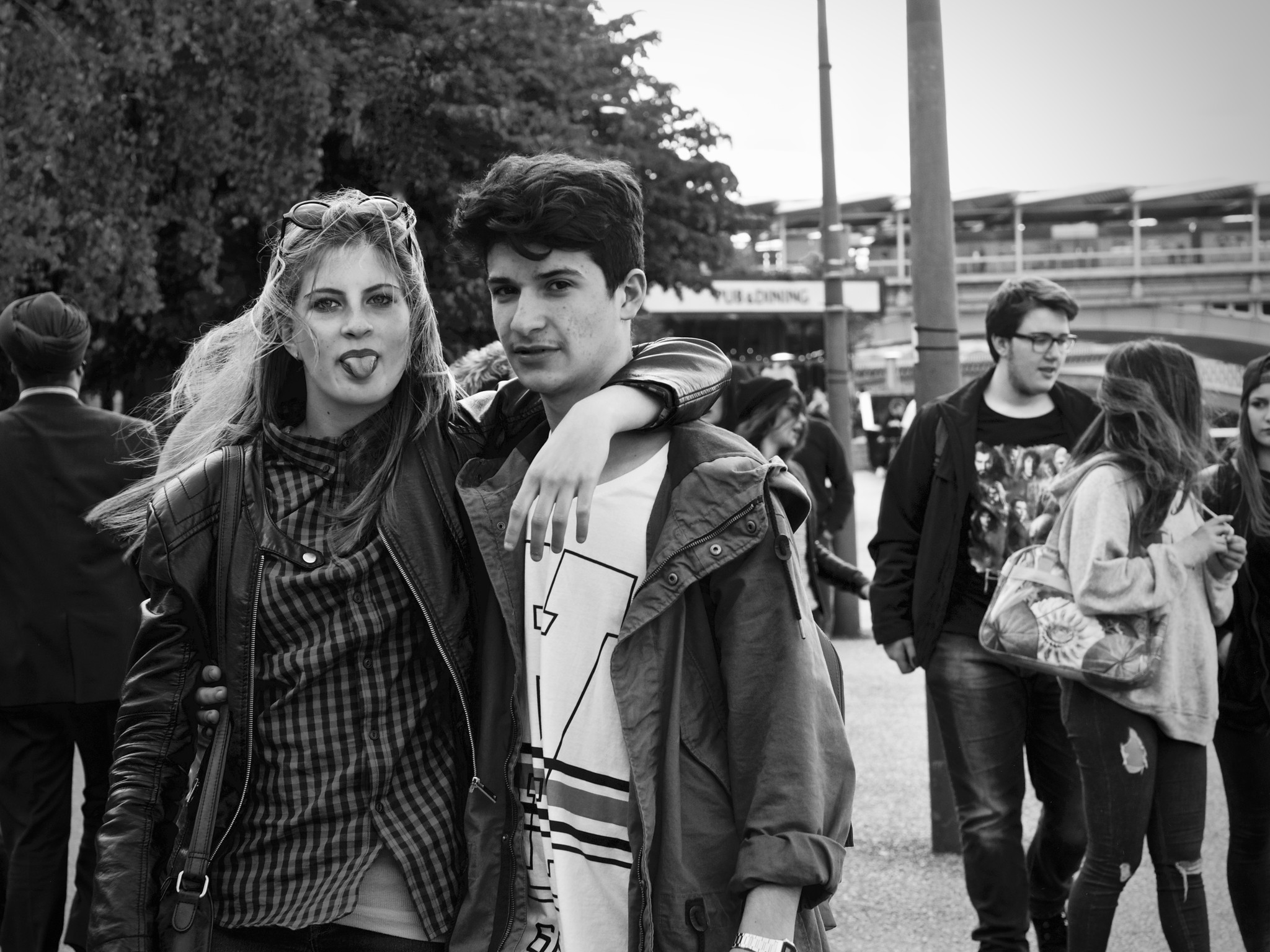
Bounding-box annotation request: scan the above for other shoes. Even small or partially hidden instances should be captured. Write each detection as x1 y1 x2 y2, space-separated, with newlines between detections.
1028 896 1067 952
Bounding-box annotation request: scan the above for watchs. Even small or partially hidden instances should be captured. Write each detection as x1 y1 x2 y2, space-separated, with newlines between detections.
731 933 798 952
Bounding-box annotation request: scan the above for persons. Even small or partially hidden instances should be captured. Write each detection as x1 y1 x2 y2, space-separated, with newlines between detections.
445 157 856 952
1203 354 1270 952
1044 337 1248 952
0 292 163 952
83 187 734 952
867 276 1104 952
722 350 874 931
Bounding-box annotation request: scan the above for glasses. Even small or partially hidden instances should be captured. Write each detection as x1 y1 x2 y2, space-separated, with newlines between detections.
781 402 801 419
999 328 1078 354
278 195 412 265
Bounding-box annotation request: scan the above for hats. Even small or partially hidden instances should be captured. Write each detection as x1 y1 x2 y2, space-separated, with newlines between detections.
1239 352 1270 405
733 375 791 425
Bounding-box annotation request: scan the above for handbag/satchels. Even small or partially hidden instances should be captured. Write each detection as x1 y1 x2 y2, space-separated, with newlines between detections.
979 462 1170 687
